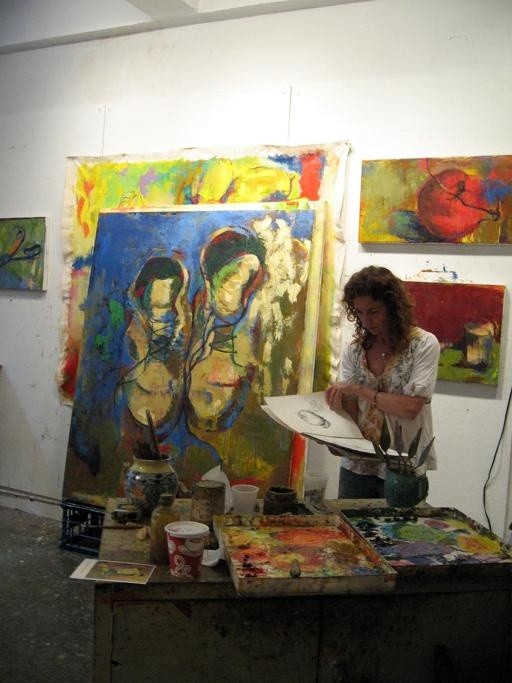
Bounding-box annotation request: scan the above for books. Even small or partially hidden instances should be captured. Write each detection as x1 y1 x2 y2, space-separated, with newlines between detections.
259 388 418 469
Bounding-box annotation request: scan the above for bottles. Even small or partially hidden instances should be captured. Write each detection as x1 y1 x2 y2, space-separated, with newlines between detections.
148 492 182 565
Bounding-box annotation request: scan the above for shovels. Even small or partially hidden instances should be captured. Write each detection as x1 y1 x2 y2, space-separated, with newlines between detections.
370 417 436 474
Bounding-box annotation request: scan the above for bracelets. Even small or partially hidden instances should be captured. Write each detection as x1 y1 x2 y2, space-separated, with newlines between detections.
373 389 379 408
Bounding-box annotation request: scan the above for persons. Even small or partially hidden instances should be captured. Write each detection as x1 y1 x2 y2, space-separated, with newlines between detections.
323 265 443 501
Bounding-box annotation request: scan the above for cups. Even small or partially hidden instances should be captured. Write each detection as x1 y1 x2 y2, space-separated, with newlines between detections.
230 484 259 514
164 520 210 582
302 470 329 505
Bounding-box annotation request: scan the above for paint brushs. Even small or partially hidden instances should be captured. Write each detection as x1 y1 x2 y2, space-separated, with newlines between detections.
89 524 145 529
132 409 167 460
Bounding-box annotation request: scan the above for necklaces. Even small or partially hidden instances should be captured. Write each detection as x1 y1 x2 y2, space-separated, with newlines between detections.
373 345 394 359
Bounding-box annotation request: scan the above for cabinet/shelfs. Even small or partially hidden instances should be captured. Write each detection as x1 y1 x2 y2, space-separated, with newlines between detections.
92 496 512 682
